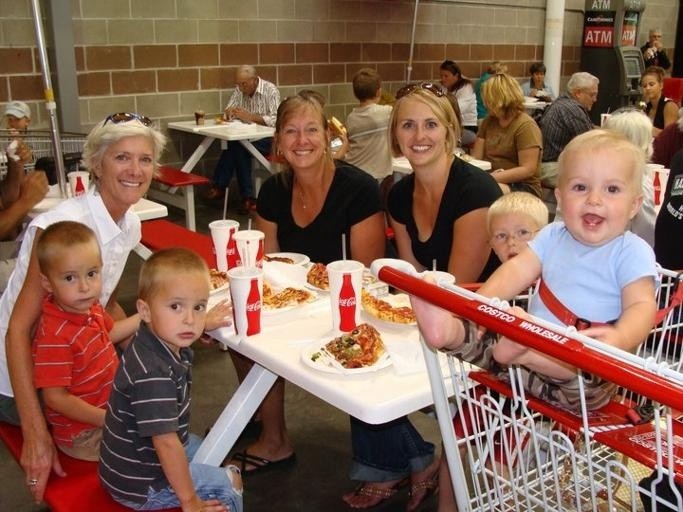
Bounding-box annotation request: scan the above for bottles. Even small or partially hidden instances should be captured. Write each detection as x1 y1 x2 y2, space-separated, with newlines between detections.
245 276 261 336
224 227 242 271
73 175 85 196
254 239 263 268
338 272 358 332
602 116 607 125
653 172 661 205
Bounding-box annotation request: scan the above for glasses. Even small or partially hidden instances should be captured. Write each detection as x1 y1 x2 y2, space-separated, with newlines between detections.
487 229 539 244
102 112 151 128
396 83 444 99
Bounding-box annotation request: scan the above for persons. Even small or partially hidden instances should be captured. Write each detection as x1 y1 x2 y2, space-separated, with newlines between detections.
0 113 168 505
339 78 508 512
340 46 682 295
297 89 350 163
254 90 387 270
406 128 657 415
29 221 147 463
200 65 281 214
638 26 672 73
0 135 48 242
4 100 35 162
97 249 244 511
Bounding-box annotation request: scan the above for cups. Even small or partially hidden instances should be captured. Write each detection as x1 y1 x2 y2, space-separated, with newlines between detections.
326 259 367 336
599 113 612 128
222 268 267 340
67 171 90 198
653 169 670 205
647 164 664 178
193 111 204 125
424 271 456 288
207 218 243 269
232 229 267 269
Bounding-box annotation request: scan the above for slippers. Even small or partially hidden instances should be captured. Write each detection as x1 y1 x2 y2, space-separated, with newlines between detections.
346 473 438 512
223 449 296 477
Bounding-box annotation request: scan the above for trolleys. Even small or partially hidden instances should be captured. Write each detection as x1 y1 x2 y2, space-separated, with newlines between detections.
0 127 90 180
369 257 682 510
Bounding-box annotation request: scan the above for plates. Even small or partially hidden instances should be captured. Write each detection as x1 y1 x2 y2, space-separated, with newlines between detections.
524 97 539 103
301 331 397 374
221 119 237 125
263 249 309 267
207 275 229 296
266 288 318 319
31 197 67 214
372 298 419 327
306 268 387 294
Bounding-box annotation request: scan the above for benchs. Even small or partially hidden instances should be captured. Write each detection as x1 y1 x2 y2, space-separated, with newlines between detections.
0 421 230 512
136 217 216 270
150 164 209 233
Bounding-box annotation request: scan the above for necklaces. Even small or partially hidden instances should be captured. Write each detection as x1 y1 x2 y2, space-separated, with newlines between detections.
294 179 307 209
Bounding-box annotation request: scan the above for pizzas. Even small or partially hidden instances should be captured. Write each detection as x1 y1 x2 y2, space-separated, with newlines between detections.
362 287 417 325
262 282 310 308
209 268 228 290
306 263 331 291
324 322 386 369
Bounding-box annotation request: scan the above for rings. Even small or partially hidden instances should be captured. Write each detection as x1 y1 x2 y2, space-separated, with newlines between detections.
30 477 36 485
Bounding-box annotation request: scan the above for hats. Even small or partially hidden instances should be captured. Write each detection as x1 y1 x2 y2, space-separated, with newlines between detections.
5 101 31 119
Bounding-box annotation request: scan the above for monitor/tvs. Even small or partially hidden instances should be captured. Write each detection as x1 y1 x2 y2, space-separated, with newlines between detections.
623 58 641 79
